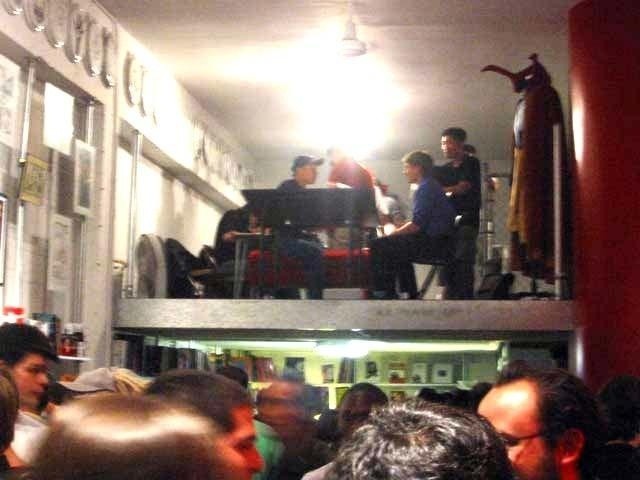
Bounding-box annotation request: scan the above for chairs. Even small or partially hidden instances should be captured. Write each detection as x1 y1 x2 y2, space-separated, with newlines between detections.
408 213 478 300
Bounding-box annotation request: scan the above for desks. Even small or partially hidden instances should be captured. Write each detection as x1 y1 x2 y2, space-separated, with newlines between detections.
230 232 274 300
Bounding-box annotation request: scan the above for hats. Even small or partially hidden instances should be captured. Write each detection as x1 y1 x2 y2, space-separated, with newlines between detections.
292 155 325 172
56 367 117 394
